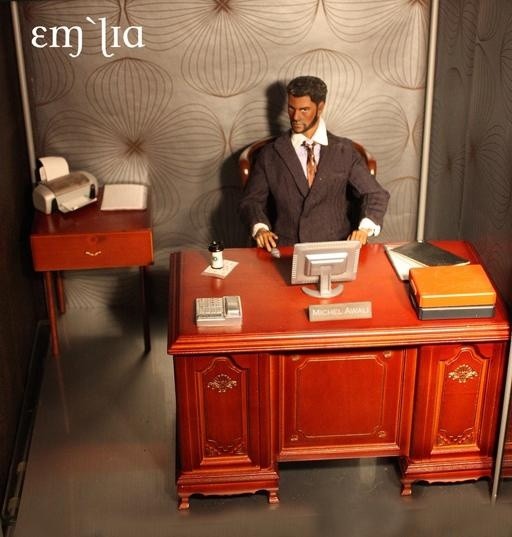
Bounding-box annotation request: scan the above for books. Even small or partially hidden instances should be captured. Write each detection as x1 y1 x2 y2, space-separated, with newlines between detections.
392 240 471 267
384 244 425 280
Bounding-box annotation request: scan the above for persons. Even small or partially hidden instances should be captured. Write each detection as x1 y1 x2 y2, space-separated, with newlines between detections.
237 76 391 249
